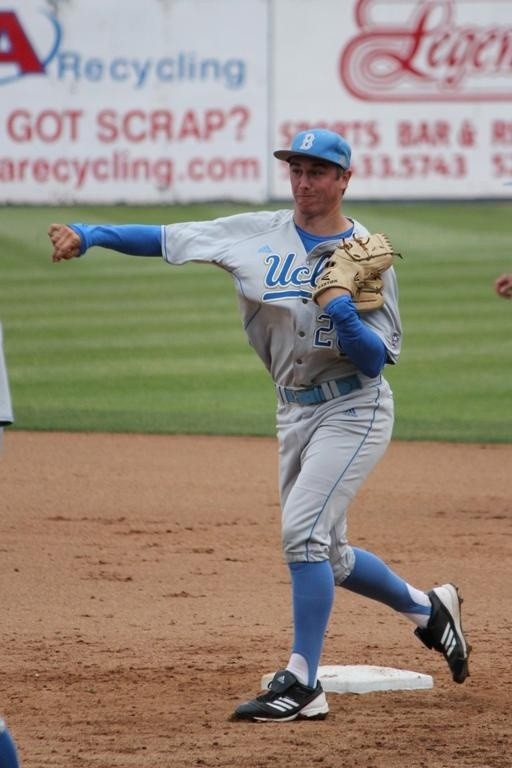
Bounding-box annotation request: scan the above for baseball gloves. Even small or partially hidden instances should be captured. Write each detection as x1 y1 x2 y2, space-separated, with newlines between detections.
312 233 403 311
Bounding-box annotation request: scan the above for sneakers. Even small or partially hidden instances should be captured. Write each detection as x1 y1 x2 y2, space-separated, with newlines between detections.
234 669 329 723
413 583 471 684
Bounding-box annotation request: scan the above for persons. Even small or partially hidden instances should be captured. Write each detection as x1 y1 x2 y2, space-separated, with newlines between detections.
0 331 19 768
48 128 471 721
494 271 512 299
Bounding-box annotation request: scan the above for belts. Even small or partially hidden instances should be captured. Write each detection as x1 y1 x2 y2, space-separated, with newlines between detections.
275 376 360 408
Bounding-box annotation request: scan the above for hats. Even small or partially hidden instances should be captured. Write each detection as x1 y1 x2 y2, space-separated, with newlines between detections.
273 128 352 171
